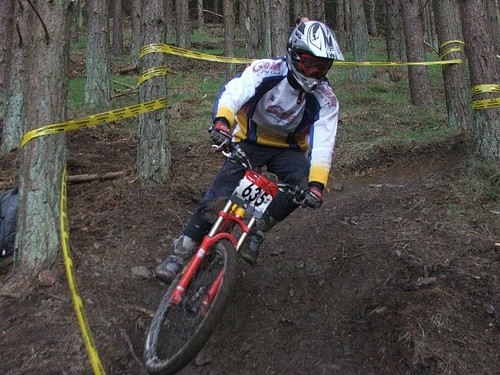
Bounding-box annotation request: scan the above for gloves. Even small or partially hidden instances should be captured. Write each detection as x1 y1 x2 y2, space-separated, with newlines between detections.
210 121 233 147
301 187 323 209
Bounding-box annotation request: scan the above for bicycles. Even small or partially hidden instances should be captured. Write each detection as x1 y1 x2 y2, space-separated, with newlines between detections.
143 125 308 375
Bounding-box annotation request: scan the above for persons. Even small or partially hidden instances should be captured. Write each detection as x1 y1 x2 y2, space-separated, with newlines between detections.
154 21 344 280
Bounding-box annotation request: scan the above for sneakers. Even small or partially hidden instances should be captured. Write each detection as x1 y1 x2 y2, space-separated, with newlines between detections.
239 215 274 267
155 234 197 285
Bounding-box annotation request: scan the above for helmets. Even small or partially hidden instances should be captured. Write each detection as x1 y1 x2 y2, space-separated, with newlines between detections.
286 20 345 95
